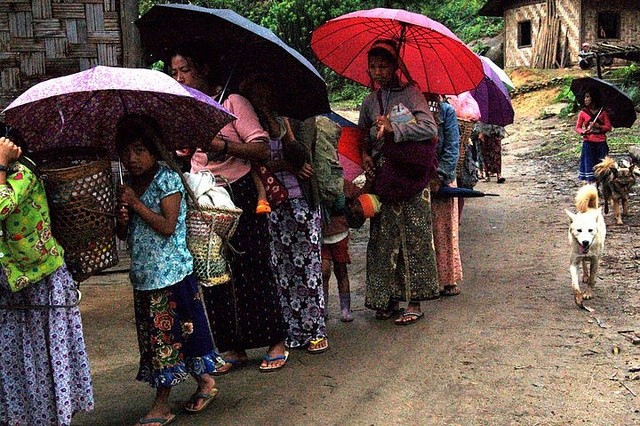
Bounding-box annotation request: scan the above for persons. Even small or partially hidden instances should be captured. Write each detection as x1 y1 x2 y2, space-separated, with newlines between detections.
474 119 505 183
168 42 290 372
114 113 226 425
357 38 440 324
427 94 463 295
238 81 287 215
259 114 329 354
575 90 611 196
321 170 376 322
0 123 93 426
472 122 495 179
577 43 594 69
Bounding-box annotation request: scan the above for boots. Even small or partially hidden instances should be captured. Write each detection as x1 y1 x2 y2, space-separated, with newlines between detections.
323 292 329 318
340 292 354 321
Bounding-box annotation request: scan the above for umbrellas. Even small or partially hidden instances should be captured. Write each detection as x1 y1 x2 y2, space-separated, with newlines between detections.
443 90 482 121
322 110 365 181
132 4 331 106
430 186 500 199
469 66 515 126
310 8 484 116
570 76 637 131
478 56 515 90
1 65 237 209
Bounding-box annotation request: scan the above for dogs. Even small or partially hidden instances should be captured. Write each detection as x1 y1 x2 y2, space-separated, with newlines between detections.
594 154 637 226
565 184 607 308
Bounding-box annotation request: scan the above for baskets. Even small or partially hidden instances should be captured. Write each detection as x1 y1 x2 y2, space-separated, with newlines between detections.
423 92 439 125
186 175 243 287
456 119 472 178
17 145 119 282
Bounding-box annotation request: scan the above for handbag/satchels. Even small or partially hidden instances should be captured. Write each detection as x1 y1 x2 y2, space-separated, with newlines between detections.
251 160 289 210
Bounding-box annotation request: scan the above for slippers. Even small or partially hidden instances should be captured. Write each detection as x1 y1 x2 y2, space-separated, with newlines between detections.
308 337 328 353
375 308 397 320
259 350 289 371
184 387 219 412
445 284 460 295
138 414 176 426
213 357 247 375
395 311 424 325
